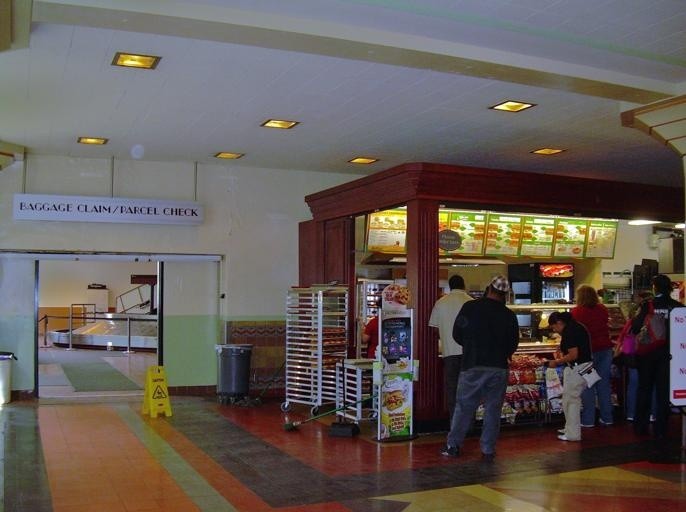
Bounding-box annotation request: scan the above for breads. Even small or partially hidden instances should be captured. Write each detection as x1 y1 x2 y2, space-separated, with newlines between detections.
322 328 347 370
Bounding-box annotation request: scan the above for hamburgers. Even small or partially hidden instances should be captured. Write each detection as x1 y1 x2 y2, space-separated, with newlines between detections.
385 391 403 411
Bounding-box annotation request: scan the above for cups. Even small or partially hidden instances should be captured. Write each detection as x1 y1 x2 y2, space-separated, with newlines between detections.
375 215 405 229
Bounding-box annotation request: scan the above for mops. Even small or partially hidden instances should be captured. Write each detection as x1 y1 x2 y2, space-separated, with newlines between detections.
240 355 286 409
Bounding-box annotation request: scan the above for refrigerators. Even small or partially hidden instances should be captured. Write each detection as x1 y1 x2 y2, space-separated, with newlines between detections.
508 261 574 336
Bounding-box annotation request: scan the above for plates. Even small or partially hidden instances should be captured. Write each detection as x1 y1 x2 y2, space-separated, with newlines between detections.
383 400 408 413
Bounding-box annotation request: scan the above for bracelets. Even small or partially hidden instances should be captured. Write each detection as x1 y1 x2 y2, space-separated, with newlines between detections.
557 359 561 365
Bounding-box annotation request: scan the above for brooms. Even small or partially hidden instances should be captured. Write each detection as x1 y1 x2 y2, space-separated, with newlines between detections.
282 391 380 432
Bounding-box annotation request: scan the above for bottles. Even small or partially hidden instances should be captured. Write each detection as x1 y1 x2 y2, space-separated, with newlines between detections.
542 281 567 300
464 241 481 252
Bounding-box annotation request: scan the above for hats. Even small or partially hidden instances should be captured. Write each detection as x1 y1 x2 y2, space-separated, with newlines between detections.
491 276 509 292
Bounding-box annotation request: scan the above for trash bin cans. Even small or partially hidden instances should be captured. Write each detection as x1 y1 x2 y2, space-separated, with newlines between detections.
214 343 253 407
0 351 17 404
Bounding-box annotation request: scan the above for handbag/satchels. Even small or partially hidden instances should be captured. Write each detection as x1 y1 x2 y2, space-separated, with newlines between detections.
579 364 602 389
634 314 667 357
621 334 636 355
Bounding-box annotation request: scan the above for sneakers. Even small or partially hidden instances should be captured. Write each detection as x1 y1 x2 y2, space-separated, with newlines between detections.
556 413 658 442
482 452 496 464
439 445 460 458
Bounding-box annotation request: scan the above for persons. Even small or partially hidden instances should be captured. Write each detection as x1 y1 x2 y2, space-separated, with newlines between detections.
541 310 592 443
632 273 683 415
615 297 661 425
569 283 617 428
426 272 478 437
438 273 520 464
360 299 383 423
